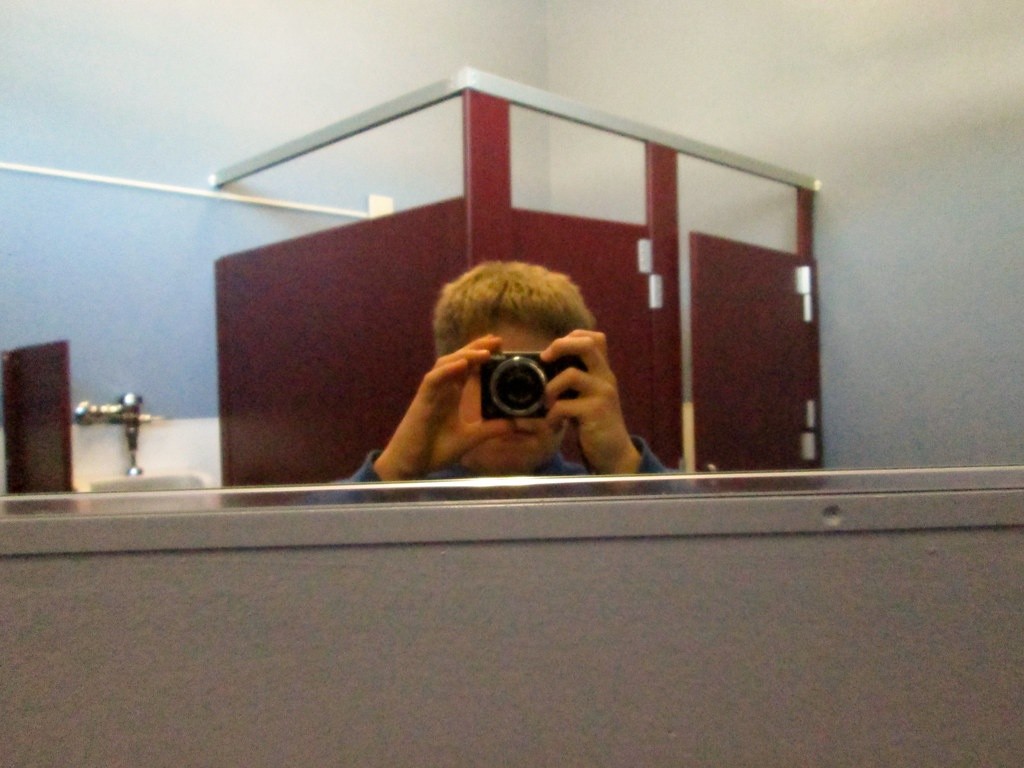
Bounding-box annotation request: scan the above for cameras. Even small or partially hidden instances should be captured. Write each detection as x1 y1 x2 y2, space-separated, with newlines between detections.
480 351 588 416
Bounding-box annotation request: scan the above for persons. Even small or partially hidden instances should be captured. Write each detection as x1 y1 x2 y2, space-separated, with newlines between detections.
307 260 703 525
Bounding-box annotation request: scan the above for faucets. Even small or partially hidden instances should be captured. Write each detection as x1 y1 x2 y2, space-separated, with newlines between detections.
74 392 146 476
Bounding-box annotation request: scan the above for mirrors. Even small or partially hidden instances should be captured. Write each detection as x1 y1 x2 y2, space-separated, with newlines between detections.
3 5 1022 491
